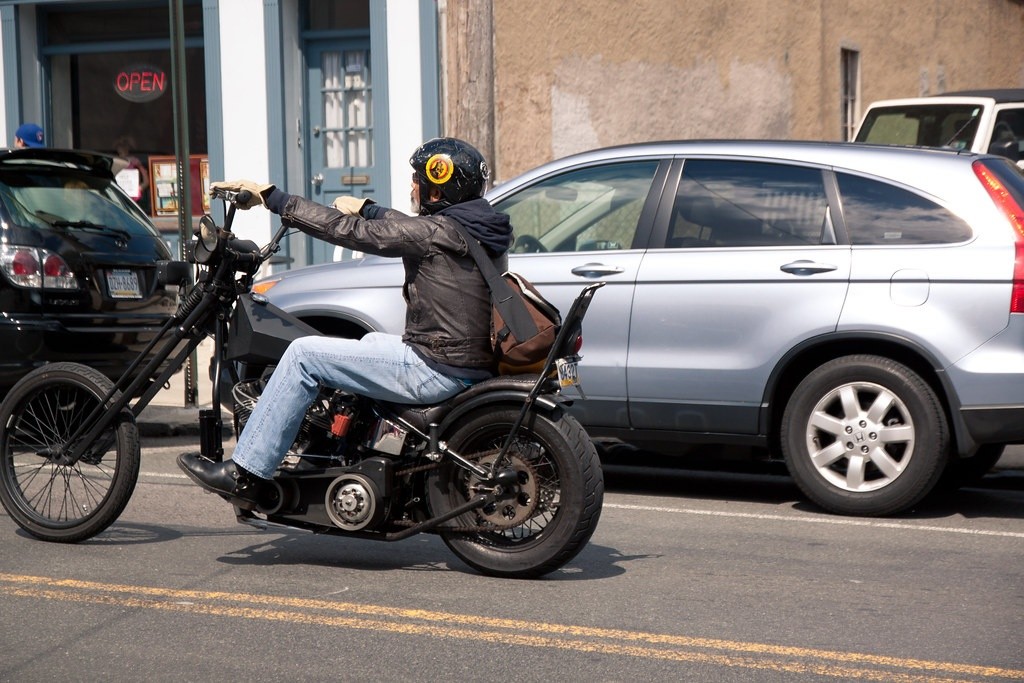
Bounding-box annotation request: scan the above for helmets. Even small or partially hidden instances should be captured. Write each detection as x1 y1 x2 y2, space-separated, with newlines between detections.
410 136 488 205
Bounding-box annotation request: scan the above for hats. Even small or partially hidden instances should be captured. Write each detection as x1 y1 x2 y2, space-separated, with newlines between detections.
16 123 44 147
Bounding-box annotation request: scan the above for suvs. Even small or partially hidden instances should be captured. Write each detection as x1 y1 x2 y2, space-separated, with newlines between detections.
1 147 179 413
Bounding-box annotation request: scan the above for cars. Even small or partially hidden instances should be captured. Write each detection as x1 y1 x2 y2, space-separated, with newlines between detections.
820 85 1024 241
220 136 1024 515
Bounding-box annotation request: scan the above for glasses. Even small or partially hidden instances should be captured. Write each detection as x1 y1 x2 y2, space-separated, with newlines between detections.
412 173 421 184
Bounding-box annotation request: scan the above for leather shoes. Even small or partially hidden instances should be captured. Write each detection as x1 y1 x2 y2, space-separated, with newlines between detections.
176 452 261 510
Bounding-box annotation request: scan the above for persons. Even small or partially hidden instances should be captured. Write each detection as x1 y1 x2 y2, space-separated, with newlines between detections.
177 138 513 512
14 125 46 148
116 137 149 202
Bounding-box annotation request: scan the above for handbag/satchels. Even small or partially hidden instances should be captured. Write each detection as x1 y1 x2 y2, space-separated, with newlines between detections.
488 271 562 378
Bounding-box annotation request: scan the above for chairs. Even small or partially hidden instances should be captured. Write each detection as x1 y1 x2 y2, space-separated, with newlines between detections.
669 182 765 249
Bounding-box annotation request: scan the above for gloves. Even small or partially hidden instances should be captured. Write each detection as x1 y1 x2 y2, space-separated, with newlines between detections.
332 195 377 221
209 180 275 209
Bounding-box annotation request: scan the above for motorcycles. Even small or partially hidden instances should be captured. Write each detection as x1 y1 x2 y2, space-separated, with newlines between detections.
0 179 611 576
